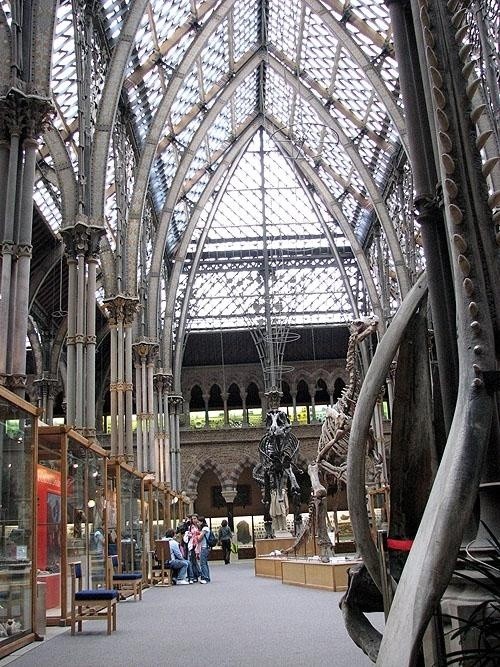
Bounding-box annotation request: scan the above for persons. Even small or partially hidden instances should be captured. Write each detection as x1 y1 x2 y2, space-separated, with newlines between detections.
94 523 105 556
160 513 216 585
218 520 232 564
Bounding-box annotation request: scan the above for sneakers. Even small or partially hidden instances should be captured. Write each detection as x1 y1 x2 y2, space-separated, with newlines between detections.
172 577 207 585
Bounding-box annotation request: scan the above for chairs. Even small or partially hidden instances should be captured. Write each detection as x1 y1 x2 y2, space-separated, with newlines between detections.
68 560 118 635
108 555 142 600
149 551 173 586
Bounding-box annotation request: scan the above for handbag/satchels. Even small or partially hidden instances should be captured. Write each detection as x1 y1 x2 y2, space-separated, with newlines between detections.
219 529 222 538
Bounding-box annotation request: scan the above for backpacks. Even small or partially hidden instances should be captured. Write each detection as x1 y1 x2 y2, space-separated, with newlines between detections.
201 526 217 546
